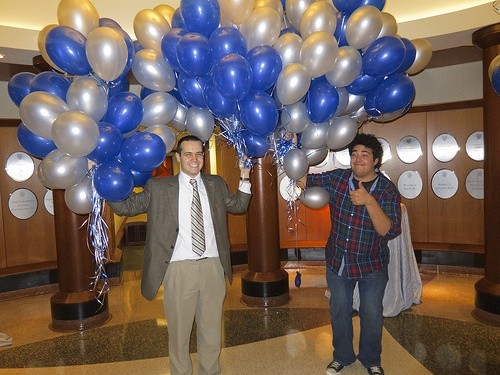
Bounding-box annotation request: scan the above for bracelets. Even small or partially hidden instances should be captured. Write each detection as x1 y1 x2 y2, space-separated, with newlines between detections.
240 177 250 181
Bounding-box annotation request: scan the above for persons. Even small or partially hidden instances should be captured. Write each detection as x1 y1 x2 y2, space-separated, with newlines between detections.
106 135 252 375
285 132 402 375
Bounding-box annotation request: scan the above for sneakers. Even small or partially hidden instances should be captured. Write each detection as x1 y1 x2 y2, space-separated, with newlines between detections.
326 360 345 375
367 365 384 375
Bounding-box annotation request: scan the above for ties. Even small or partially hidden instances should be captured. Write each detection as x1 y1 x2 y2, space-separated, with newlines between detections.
189 179 206 257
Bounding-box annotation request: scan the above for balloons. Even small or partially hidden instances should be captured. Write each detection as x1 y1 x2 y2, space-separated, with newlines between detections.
488 54 500 95
299 186 330 209
7 0 435 188
42 149 88 187
94 161 134 200
64 172 100 215
